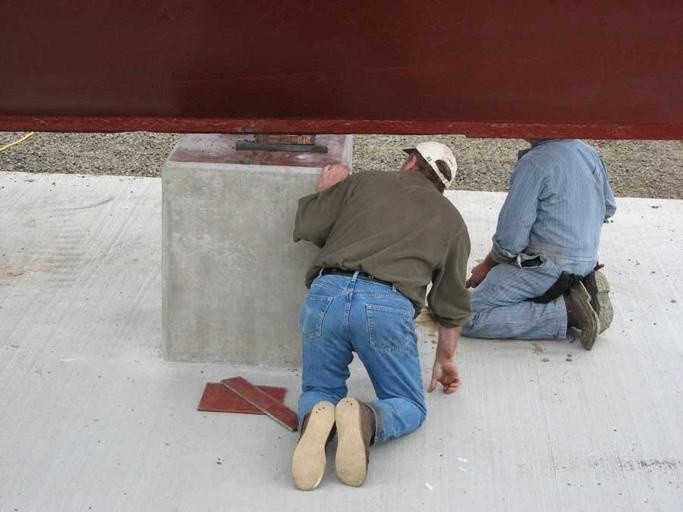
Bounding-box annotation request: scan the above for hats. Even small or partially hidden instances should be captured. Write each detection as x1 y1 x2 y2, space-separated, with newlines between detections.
404 142 457 190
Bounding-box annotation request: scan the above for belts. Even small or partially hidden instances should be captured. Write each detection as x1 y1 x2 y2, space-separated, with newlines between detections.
322 265 391 286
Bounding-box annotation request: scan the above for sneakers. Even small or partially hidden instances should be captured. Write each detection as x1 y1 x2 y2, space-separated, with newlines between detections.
333 396 372 486
565 278 601 350
291 400 336 489
583 270 612 334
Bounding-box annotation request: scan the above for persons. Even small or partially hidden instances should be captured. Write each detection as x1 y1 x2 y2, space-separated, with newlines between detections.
288 139 472 493
457 135 621 351
